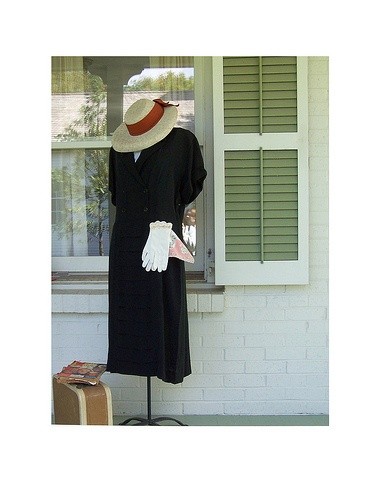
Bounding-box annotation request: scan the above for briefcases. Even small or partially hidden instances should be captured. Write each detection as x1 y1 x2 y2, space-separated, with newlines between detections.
52 373 113 425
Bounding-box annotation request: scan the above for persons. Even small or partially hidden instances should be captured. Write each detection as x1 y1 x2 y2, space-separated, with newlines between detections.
106 98 207 384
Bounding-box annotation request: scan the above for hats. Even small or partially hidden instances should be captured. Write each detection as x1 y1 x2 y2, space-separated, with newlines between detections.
112 99 179 152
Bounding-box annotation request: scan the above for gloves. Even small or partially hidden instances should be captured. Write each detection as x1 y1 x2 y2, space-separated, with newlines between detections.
142 221 172 272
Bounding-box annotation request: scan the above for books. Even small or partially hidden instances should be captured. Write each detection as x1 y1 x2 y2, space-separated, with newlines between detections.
57 359 109 385
